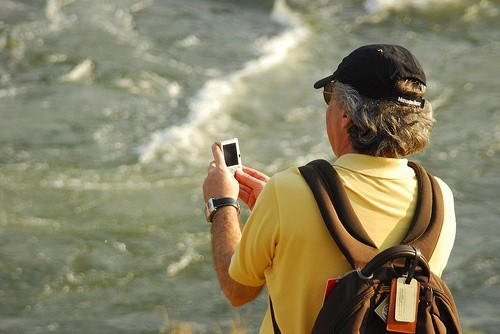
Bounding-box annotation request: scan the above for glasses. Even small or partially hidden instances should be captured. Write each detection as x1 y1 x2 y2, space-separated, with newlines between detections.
323 82 337 105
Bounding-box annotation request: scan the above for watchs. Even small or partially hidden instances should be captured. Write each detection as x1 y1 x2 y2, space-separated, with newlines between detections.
204 197 240 223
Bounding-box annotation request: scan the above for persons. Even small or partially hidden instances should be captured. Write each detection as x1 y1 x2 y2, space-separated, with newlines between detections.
202 43 457 334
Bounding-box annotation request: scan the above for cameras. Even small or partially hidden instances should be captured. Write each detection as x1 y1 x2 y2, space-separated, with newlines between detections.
214 138 243 176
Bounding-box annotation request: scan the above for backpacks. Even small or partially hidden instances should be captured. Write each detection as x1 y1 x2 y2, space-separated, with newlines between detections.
298 159 464 334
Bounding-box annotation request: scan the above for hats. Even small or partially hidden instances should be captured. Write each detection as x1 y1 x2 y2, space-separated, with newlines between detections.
313 44 427 109
